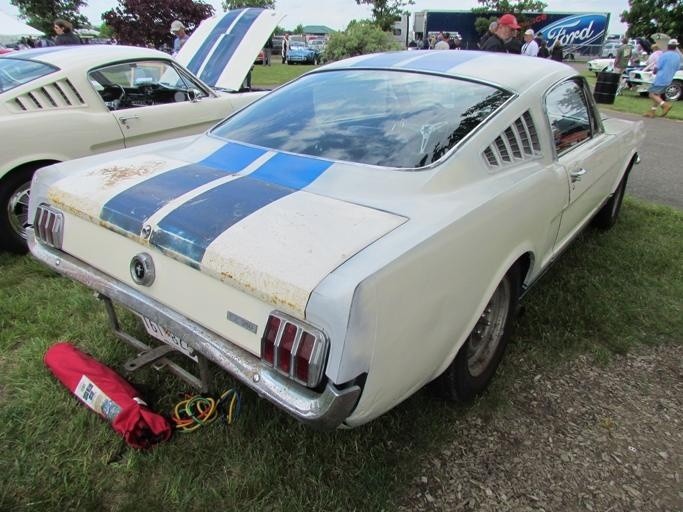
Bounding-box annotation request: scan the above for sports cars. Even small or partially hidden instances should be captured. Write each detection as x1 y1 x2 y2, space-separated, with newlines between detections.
21 48 645 436
2 7 288 267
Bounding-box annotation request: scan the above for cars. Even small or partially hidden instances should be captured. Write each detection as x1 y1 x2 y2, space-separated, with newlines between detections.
253 32 329 66
0 32 25 54
588 31 682 106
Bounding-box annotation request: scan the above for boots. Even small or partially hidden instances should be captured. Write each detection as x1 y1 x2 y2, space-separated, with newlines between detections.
642 109 656 118
657 102 673 118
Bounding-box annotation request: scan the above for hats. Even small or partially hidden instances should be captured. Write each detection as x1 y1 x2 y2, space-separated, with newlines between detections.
498 14 522 29
668 38 680 45
525 29 535 36
171 19 185 32
454 35 466 40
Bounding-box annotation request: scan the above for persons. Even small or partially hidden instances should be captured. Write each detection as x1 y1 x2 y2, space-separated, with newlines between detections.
262 36 273 66
480 14 562 62
15 35 58 49
643 39 680 117
170 20 189 53
613 38 663 96
419 33 460 50
55 20 83 45
282 33 290 64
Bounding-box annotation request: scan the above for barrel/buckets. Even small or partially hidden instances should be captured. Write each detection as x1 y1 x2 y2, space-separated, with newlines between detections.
593 72 621 104
